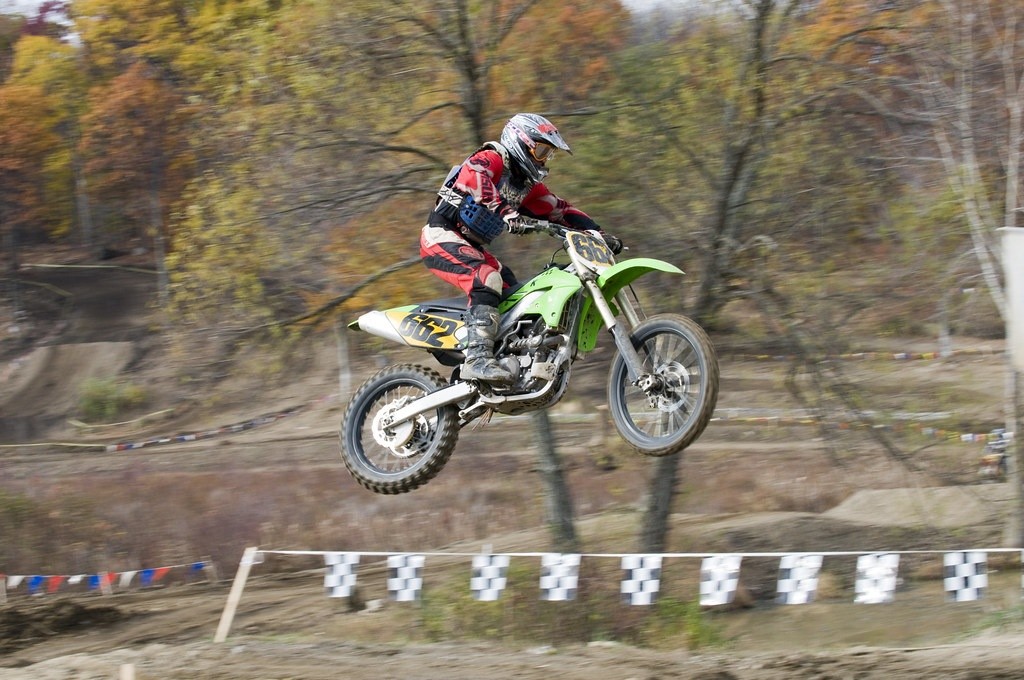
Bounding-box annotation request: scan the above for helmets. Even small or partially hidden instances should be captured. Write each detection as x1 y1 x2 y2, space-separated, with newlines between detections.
500 113 573 186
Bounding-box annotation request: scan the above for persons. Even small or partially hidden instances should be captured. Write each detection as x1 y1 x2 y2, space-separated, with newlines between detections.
418 111 622 382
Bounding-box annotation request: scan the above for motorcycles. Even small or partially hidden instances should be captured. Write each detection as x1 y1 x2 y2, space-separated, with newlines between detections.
338 215 722 496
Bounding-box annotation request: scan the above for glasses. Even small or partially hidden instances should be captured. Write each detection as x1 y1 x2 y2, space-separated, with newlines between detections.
528 142 558 161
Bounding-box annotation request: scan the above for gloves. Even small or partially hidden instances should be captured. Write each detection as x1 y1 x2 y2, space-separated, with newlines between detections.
500 209 526 236
600 230 623 255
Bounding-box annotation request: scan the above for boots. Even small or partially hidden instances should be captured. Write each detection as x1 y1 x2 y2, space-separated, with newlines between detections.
459 305 514 385
